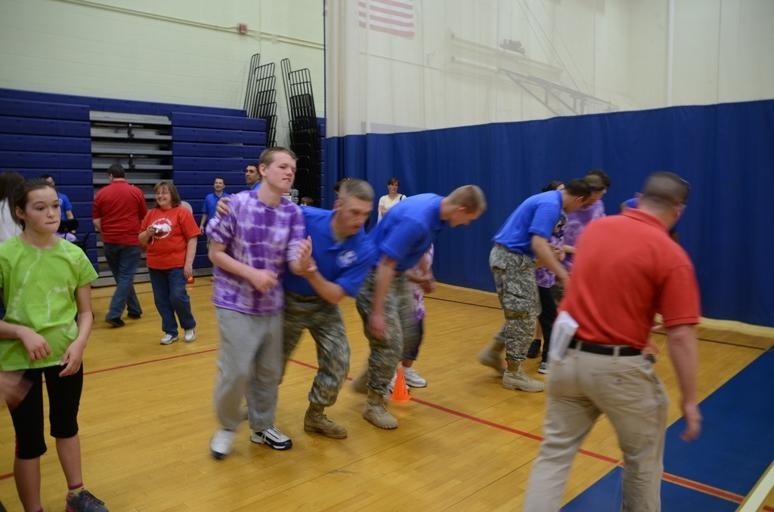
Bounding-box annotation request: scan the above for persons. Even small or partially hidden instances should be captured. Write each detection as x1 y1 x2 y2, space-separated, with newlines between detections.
216 177 374 439
377 177 406 224
527 170 702 512
199 176 230 283
353 184 487 429
528 170 610 358
40 174 77 241
387 244 435 393
479 179 591 395
92 164 148 326
0 170 24 245
0 179 107 512
138 180 200 346
245 163 261 190
620 197 677 330
535 182 580 373
207 146 316 458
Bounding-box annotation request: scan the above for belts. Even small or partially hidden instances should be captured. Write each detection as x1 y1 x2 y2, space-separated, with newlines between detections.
569 337 641 356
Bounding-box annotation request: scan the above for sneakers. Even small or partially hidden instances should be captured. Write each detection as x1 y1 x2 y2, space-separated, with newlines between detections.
527 339 541 358
106 317 124 326
364 389 398 429
185 328 195 341
304 403 347 438
403 366 426 388
502 369 544 392
160 334 178 344
480 348 506 374
66 491 109 511
537 362 547 374
127 312 140 319
355 371 368 393
251 425 292 450
211 428 233 459
